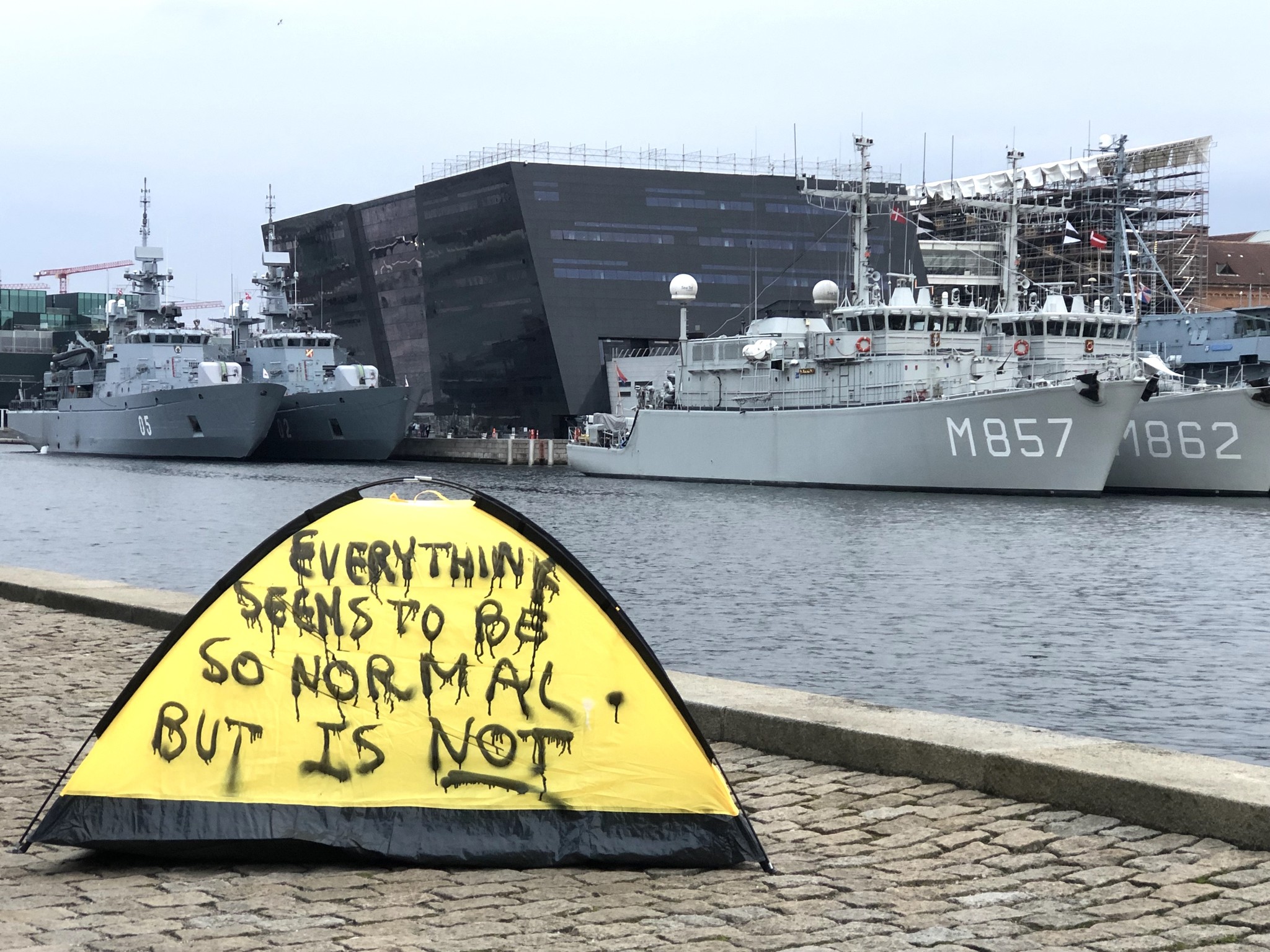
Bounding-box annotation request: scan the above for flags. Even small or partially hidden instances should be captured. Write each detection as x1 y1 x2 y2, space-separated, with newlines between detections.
1137 281 1151 304
1090 230 1108 249
118 288 123 296
245 292 251 300
616 365 628 383
889 205 907 224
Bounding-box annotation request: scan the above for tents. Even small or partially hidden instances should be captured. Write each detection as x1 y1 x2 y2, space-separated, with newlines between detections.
18 474 775 878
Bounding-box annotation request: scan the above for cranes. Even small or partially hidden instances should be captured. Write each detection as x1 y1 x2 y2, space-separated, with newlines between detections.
34 259 135 295
0 272 51 290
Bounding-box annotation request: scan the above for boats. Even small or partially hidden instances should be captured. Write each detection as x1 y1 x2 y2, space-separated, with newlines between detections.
565 109 1270 501
203 183 425 465
4 177 286 464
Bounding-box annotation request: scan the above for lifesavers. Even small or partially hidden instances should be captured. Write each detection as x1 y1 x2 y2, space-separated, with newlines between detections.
855 336 871 352
1014 339 1029 355
